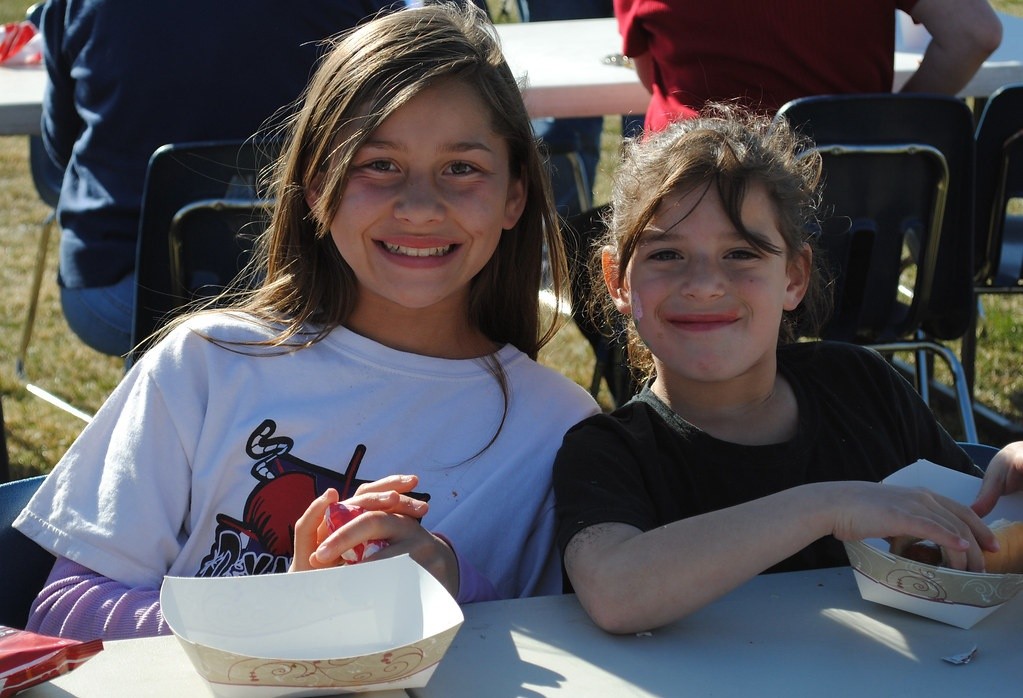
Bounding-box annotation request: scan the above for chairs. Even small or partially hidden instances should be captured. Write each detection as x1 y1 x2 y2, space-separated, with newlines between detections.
16 78 1023 475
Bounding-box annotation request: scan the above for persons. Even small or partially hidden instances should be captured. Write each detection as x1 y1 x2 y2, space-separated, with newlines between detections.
552 102 1023 633
12 0 600 642
29 0 1003 364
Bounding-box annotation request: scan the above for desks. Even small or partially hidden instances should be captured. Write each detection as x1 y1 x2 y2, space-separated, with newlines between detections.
0 8 1023 133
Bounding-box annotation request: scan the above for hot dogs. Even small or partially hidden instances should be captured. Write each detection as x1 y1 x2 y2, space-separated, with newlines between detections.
886 515 1023 575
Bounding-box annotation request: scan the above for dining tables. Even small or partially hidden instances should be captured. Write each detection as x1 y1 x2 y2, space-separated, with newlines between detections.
12 566 1023 698
